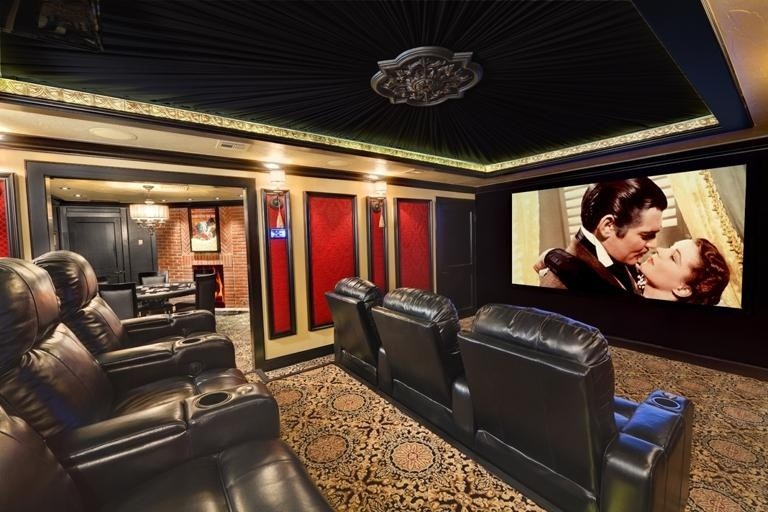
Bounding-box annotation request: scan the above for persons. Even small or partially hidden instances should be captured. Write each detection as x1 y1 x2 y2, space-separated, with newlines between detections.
531 237 731 309
535 176 670 299
192 216 216 241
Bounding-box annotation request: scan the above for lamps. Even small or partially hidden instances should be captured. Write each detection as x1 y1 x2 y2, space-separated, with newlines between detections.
128 185 169 238
263 165 289 209
369 181 387 212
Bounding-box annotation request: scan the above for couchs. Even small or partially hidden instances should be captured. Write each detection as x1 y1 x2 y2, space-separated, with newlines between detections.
1 254 286 444
325 278 388 400
453 292 690 512
32 252 223 349
370 283 473 461
0 383 332 512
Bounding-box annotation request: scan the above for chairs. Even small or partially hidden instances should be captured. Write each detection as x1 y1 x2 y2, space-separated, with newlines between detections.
97 270 216 321
